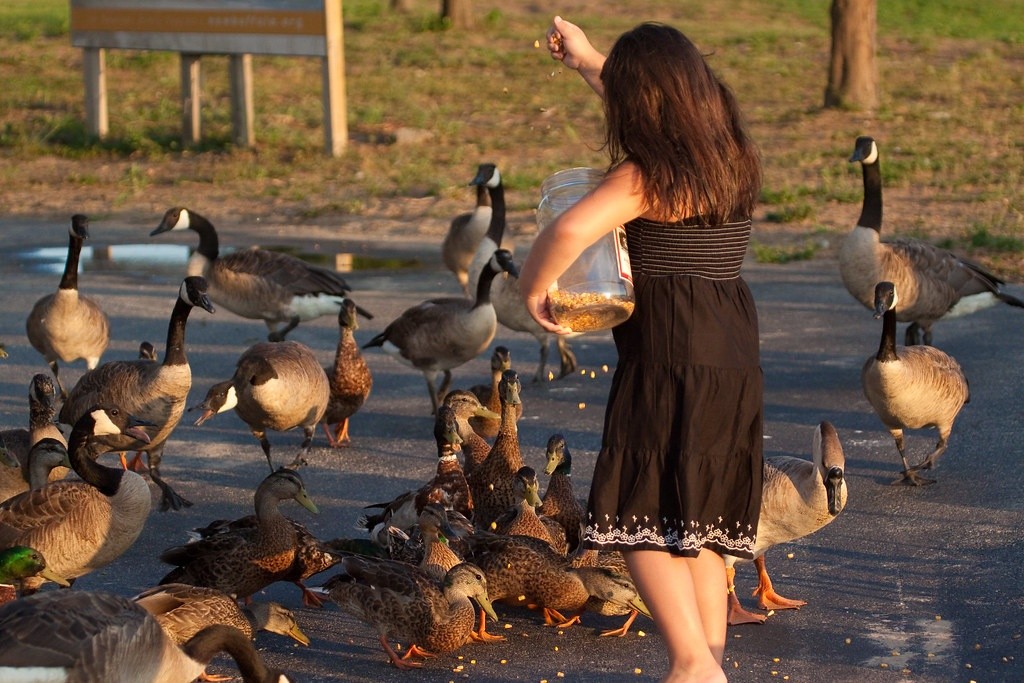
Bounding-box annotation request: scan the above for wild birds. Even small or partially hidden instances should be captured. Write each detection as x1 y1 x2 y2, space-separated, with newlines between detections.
860 281 972 488
2 161 654 683
837 136 1024 346
722 419 848 625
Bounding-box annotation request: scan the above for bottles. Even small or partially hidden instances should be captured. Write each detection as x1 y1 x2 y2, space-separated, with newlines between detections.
534 169 636 334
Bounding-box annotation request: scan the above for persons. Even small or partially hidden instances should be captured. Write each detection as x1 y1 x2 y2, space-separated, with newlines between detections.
517 16 765 683
